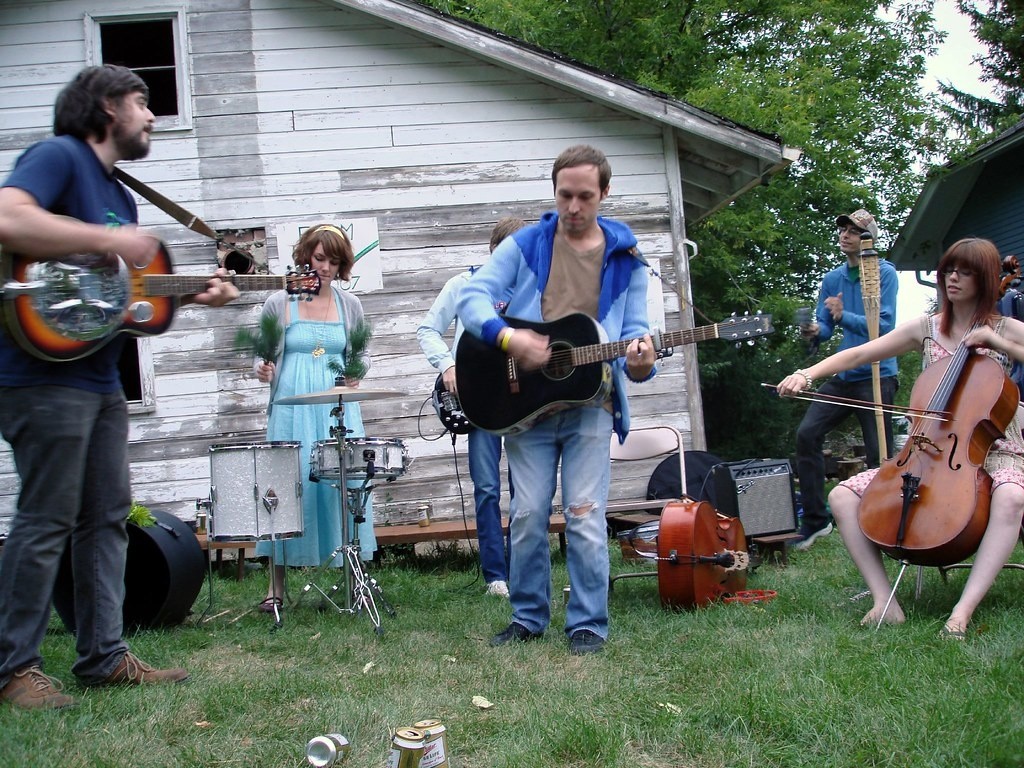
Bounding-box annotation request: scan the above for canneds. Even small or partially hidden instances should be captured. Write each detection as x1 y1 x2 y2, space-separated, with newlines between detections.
386 719 449 768
304 733 350 768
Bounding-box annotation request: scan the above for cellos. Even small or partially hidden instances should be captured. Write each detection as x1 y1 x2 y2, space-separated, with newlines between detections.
856 255 1024 634
655 499 764 612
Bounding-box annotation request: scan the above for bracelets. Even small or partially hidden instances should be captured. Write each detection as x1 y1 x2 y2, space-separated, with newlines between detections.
794 370 811 390
501 327 515 349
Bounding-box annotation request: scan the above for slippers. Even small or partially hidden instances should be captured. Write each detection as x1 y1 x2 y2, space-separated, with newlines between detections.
935 627 966 645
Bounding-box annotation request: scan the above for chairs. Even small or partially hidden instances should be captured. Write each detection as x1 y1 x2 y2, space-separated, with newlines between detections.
873 401 1024 600
607 426 698 597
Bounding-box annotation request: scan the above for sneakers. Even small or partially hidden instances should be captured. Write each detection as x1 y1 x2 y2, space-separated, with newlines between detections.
93 651 188 686
485 580 509 598
489 622 543 647
0 664 74 712
790 521 833 552
569 629 604 656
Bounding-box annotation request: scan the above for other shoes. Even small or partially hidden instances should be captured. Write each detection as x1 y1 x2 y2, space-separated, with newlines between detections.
352 593 372 608
258 596 283 612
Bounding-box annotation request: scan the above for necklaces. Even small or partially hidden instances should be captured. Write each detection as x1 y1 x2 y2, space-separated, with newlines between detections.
305 291 332 357
951 329 957 350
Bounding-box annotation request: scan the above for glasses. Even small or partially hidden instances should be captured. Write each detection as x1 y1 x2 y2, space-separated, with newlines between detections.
838 225 860 236
942 264 978 277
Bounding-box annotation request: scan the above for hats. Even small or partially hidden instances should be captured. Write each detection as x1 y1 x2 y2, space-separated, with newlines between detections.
836 208 878 245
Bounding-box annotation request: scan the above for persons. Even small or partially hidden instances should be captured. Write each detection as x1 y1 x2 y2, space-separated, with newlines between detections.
418 218 532 597
0 65 238 708
776 238 1024 642
796 208 899 553
454 145 657 653
254 223 378 611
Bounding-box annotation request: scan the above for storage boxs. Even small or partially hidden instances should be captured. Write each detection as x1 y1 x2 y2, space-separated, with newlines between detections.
208 441 304 542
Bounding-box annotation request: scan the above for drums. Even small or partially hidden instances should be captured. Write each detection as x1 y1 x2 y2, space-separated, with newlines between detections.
310 435 405 481
209 440 304 543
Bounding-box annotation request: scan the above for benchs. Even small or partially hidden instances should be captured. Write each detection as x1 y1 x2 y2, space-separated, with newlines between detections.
194 514 565 580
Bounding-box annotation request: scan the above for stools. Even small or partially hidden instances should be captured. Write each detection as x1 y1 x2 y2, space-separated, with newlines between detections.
750 533 804 568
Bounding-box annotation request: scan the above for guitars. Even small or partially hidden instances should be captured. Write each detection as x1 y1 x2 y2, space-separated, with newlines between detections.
1 234 322 364
454 309 775 438
431 371 478 435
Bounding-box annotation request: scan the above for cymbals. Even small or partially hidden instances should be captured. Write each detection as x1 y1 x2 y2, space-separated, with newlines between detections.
273 385 410 406
